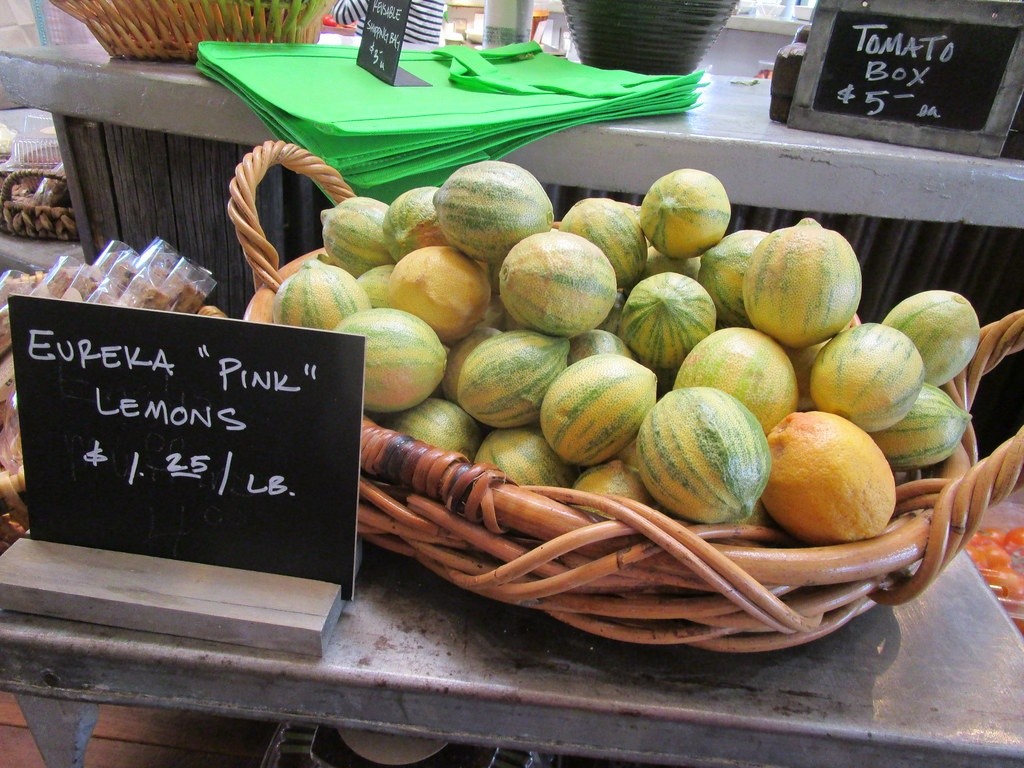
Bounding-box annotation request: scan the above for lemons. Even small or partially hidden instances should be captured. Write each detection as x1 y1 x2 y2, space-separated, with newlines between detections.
269 161 980 545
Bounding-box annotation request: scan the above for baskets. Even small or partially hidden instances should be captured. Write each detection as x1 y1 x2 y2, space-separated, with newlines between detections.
226 139 1023 653
48 1 337 59
0 170 78 240
1 272 228 540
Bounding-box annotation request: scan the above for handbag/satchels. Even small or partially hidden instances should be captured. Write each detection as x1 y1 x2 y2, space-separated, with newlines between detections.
195 39 711 207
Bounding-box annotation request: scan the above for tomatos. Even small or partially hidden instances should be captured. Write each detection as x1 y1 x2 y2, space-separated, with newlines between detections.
963 526 1024 641
